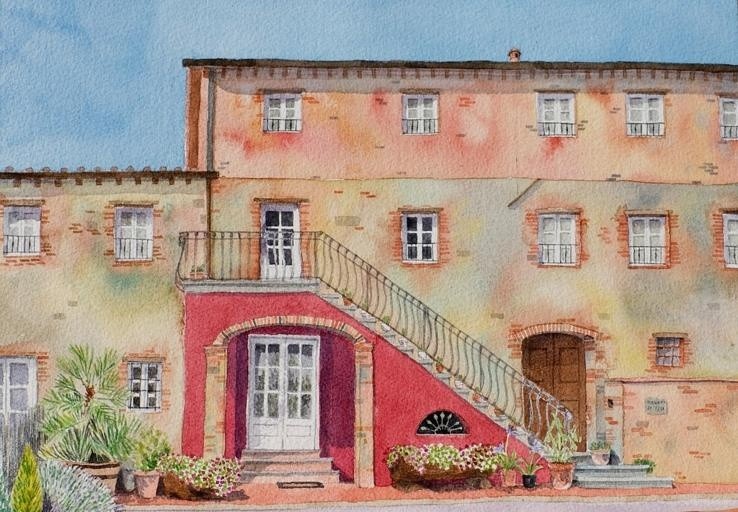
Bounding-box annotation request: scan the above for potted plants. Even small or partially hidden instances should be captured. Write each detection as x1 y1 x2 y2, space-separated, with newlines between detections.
588 439 611 464
32 343 170 498
514 407 579 490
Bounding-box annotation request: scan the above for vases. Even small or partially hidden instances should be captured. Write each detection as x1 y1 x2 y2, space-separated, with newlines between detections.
502 469 517 487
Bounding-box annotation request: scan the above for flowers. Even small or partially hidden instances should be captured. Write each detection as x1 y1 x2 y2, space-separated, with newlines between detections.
157 452 244 500
381 439 502 479
496 423 519 469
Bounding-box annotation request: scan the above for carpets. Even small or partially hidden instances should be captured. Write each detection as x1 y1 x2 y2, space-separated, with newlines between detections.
277 479 326 489
